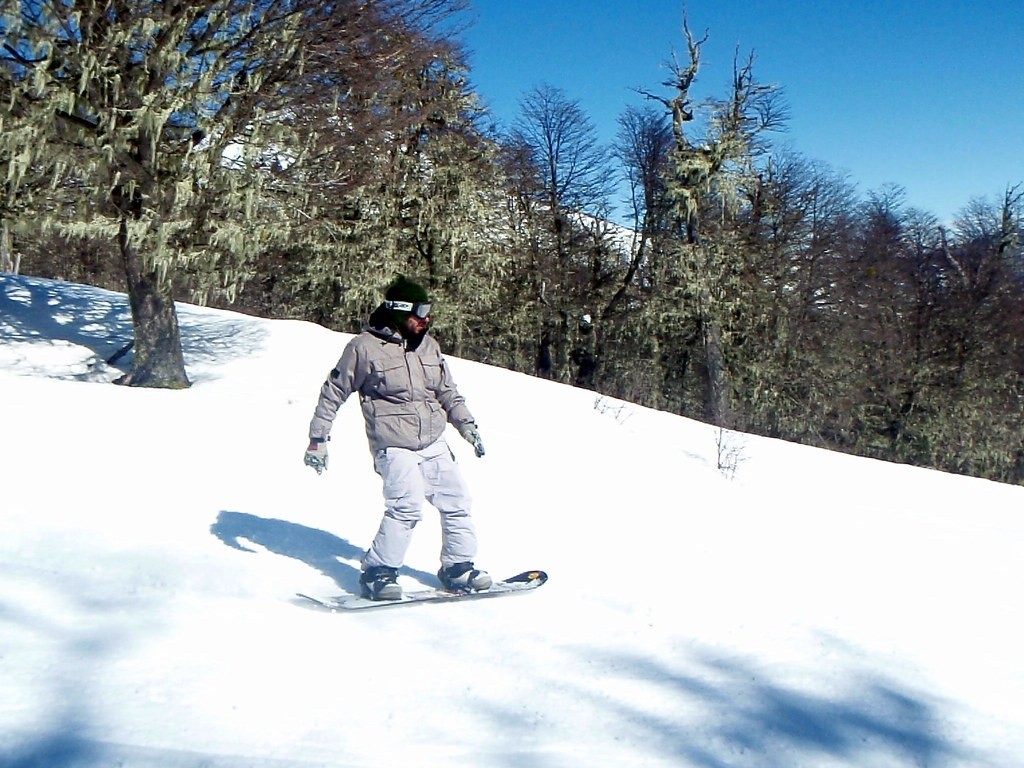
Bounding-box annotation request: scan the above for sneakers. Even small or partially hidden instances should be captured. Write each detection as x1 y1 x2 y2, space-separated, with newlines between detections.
358 566 402 600
438 562 492 590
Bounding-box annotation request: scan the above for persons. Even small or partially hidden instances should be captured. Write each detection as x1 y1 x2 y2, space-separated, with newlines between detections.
304 275 491 600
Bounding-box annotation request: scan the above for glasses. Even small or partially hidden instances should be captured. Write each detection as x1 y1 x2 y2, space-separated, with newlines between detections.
414 301 432 319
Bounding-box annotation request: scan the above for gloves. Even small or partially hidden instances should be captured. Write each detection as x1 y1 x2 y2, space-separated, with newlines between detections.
458 423 485 458
303 441 329 475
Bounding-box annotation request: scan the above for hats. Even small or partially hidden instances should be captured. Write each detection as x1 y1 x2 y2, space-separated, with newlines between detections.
581 314 592 323
384 275 428 311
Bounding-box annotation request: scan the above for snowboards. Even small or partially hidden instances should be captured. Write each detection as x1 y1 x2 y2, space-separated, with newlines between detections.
295 569 550 613
